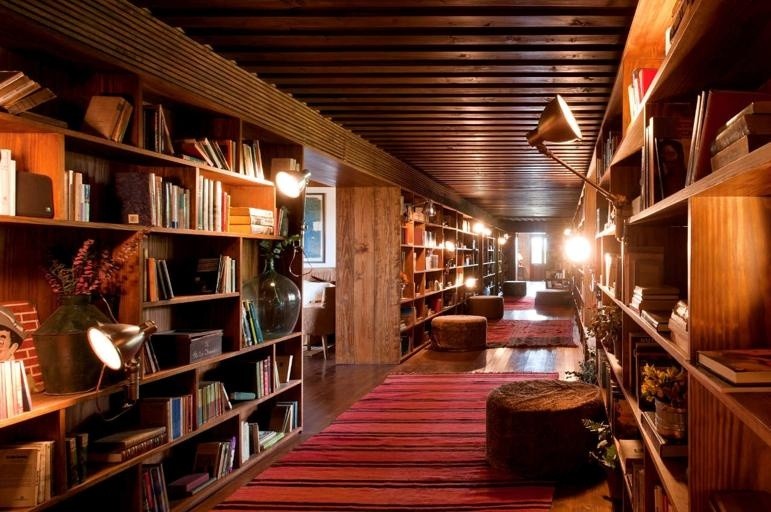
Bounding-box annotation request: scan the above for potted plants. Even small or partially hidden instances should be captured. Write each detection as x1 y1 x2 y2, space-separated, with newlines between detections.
242 234 301 337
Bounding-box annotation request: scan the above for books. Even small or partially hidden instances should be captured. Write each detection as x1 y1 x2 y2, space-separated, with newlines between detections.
190 254 235 294
141 433 237 512
242 139 300 180
198 355 293 426
146 103 236 172
242 400 298 462
630 284 689 352
1 441 58 507
65 169 91 221
627 0 771 206
143 341 159 374
241 301 263 347
64 394 193 484
690 349 771 394
593 131 640 439
715 491 771 512
1 70 134 142
0 359 32 418
143 247 174 302
0 148 16 216
616 411 689 512
116 172 272 235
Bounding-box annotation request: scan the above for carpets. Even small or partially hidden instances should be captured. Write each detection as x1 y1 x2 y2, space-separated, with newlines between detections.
504 294 535 310
486 319 578 349
303 344 334 357
207 372 559 511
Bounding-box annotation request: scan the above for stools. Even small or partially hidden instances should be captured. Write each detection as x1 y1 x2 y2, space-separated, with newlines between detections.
487 381 604 478
536 291 569 308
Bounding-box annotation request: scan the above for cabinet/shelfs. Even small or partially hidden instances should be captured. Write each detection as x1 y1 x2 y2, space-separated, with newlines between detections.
564 0 771 512
0 10 305 512
335 185 509 364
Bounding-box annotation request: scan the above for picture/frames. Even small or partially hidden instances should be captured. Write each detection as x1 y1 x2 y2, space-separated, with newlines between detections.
303 193 325 264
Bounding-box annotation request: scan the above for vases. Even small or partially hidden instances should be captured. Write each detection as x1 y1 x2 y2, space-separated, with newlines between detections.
31 295 114 395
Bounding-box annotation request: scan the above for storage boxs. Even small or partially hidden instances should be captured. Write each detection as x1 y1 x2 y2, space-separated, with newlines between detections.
150 330 223 365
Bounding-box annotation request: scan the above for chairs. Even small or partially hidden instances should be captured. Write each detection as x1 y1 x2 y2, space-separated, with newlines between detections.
304 282 336 360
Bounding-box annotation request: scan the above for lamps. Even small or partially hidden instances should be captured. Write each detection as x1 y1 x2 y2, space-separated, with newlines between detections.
275 168 311 199
525 94 632 244
87 320 158 423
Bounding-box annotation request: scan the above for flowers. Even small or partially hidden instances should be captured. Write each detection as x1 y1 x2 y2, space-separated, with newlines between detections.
38 224 153 296
